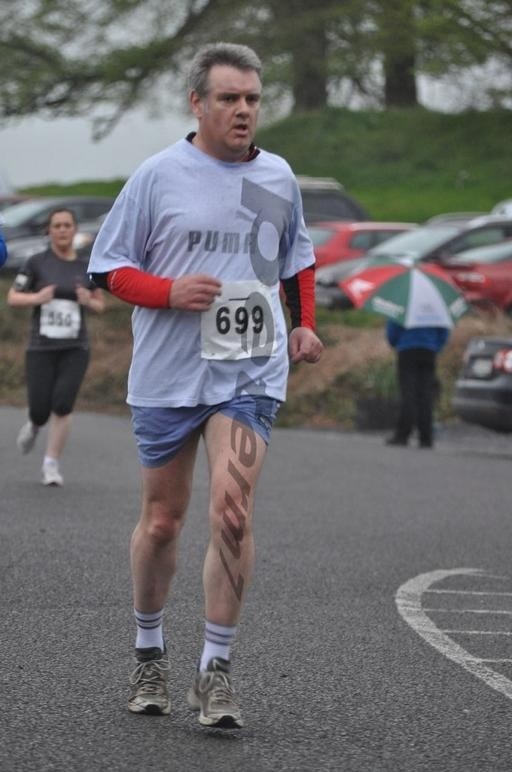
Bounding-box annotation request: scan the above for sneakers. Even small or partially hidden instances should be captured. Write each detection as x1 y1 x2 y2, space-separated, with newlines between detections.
187 655 245 728
40 460 64 487
385 437 432 448
18 420 38 456
127 637 173 714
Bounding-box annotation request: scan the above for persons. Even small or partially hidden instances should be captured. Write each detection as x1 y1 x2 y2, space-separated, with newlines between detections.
84 39 327 727
8 209 109 488
382 316 446 450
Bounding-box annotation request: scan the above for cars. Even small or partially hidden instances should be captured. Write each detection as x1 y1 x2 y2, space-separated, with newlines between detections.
0 193 116 278
452 337 511 433
295 177 512 315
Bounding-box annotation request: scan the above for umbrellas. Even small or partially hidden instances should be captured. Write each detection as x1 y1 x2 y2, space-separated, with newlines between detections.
333 250 470 333
